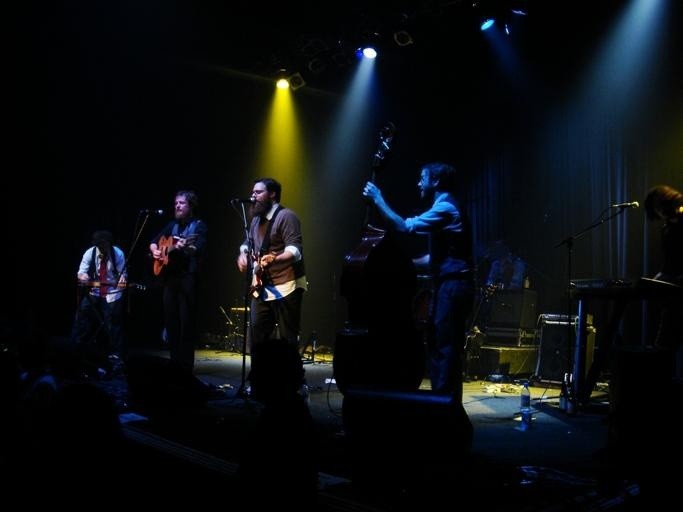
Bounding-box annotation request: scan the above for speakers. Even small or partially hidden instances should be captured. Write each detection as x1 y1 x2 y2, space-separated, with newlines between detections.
535 317 582 389
340 384 473 455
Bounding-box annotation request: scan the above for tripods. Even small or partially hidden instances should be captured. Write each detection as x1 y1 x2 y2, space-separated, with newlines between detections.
229 204 261 416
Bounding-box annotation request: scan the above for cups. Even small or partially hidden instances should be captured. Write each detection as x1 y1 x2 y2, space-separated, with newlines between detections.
560 381 576 417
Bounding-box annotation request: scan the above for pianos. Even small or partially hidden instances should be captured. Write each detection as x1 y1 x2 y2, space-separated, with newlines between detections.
569 279 681 296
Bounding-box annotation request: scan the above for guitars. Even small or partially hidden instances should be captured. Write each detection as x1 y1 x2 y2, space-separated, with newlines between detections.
78 275 146 294
249 252 278 293
154 235 198 275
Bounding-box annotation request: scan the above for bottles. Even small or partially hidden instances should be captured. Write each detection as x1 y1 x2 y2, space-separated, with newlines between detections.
104 367 129 397
520 383 530 412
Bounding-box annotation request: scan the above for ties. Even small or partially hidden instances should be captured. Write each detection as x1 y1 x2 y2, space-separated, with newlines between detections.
100 254 107 297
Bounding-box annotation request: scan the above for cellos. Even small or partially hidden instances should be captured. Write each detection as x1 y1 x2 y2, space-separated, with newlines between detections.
334 122 427 392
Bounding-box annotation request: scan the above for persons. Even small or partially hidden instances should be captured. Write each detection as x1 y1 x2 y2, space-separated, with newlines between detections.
362 162 477 439
146 190 209 375
645 185 683 283
235 178 309 403
76 231 128 349
484 240 527 288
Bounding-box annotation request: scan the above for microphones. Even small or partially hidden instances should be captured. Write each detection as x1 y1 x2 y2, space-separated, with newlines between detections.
378 120 396 139
233 196 258 203
140 207 167 217
614 198 641 210
